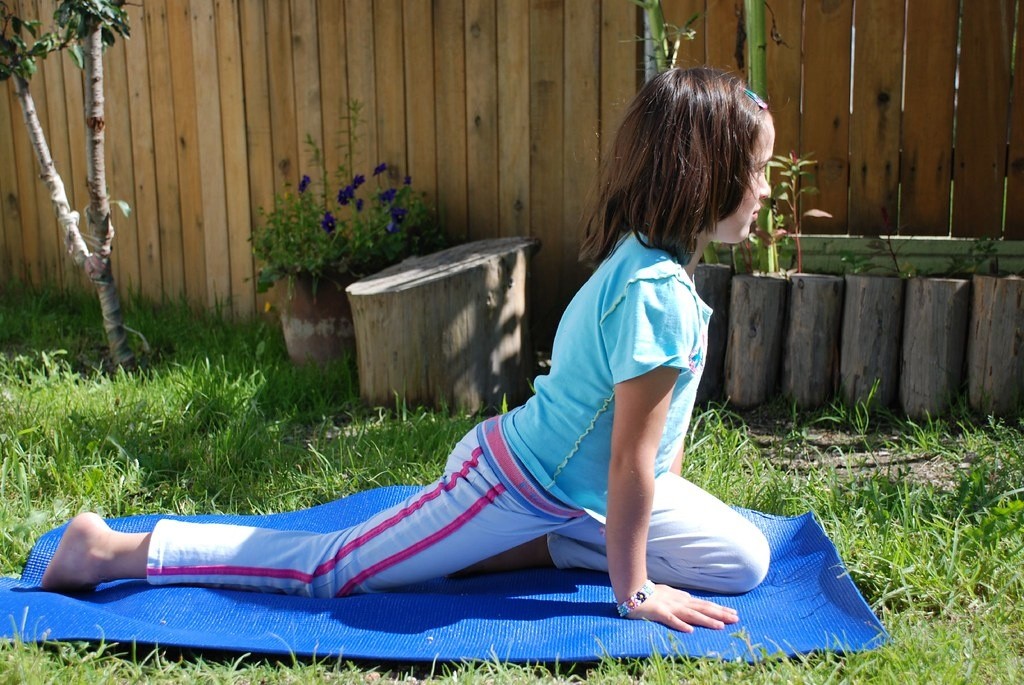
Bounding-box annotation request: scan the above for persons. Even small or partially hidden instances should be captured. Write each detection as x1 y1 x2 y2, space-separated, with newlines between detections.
39 66 775 633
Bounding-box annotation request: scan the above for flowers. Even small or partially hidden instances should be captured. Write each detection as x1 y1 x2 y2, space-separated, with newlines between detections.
248 99 445 314
768 150 834 273
855 207 914 278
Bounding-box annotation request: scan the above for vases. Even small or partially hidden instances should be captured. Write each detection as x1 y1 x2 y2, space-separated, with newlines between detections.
273 265 357 369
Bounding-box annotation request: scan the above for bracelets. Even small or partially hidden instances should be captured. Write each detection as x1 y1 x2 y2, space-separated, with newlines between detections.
616 580 655 617
